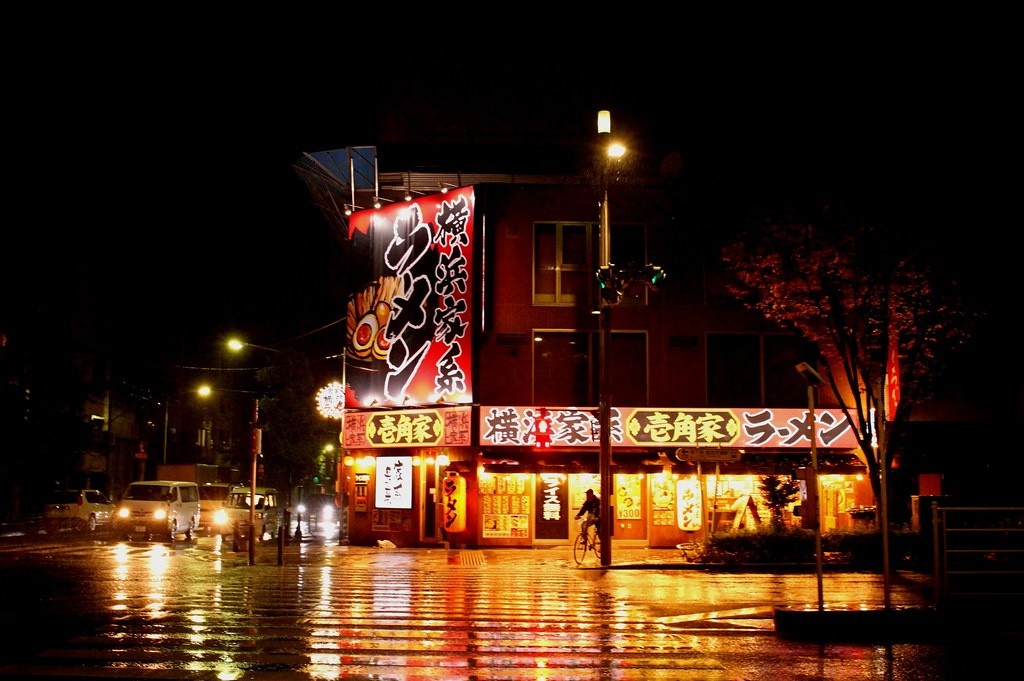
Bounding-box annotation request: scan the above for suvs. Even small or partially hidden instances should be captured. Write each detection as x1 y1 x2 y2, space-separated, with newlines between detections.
42 488 117 537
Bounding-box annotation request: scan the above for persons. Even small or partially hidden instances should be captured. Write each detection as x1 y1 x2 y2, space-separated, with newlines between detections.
255 498 269 510
574 489 600 553
239 498 248 506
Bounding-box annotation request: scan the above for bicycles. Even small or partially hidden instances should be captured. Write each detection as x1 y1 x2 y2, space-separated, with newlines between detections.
574 517 602 564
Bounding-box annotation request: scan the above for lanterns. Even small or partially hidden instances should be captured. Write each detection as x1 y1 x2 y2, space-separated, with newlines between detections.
677 480 702 533
443 472 466 534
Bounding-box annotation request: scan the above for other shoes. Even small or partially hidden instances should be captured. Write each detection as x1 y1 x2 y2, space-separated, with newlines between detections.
580 541 587 545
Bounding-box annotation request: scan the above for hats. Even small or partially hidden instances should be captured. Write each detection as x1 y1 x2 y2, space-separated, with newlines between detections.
584 489 593 494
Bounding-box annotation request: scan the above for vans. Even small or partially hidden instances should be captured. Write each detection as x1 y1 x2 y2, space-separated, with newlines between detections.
220 488 277 541
196 483 246 535
114 480 200 543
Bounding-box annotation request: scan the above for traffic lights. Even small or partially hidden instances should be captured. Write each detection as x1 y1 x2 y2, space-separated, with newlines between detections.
647 264 676 300
598 264 623 308
251 429 260 455
313 475 321 485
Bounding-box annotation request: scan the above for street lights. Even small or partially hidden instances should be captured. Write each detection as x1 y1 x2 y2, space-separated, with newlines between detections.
225 337 347 509
796 360 830 609
591 105 627 564
194 384 261 567
